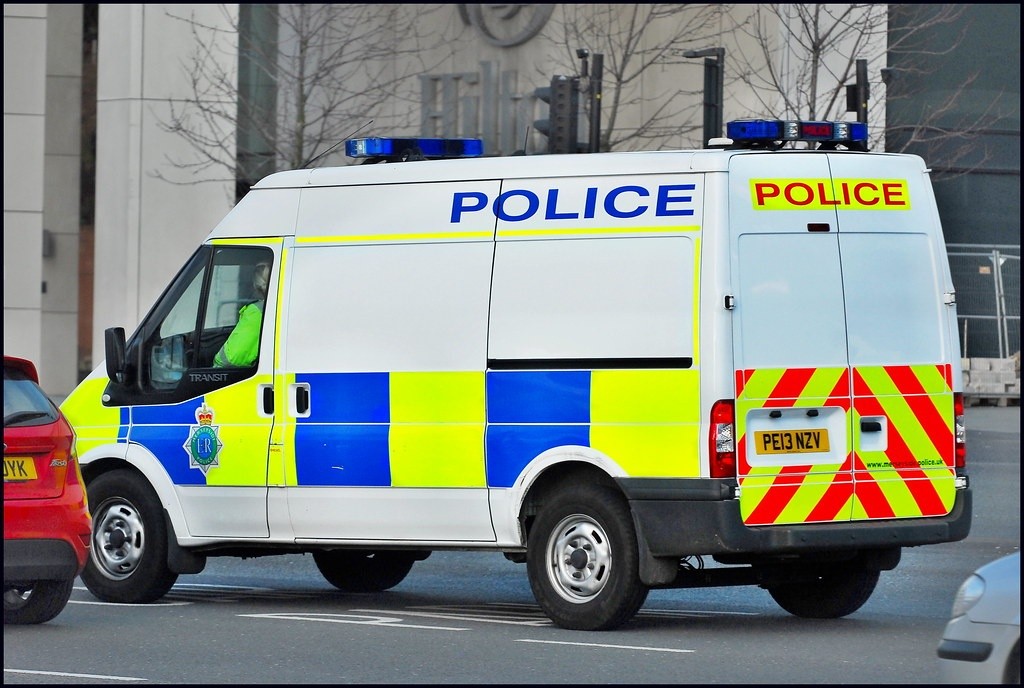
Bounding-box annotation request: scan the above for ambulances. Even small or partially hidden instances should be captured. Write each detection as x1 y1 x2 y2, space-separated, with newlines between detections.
57 120 976 629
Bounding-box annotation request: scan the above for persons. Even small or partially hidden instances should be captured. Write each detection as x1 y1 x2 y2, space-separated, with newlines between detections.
214 262 271 368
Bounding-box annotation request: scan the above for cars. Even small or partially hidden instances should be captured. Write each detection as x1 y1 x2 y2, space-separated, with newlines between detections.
3 354 95 627
934 550 1021 685
